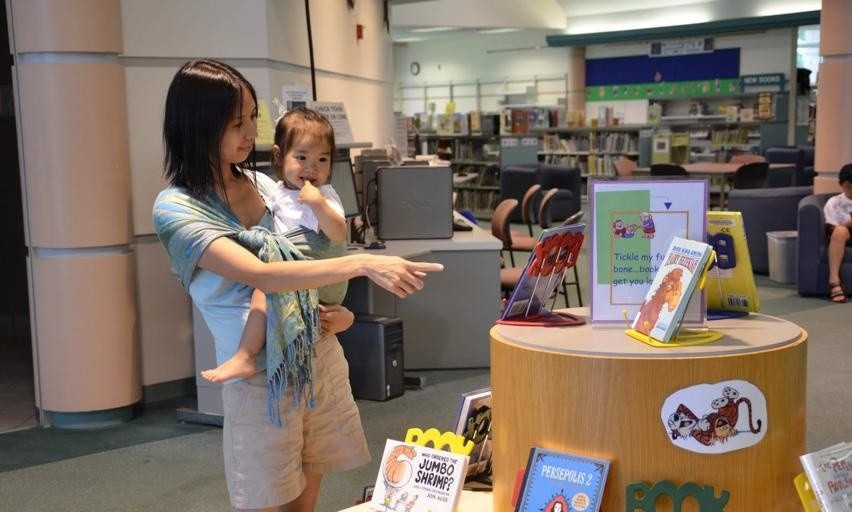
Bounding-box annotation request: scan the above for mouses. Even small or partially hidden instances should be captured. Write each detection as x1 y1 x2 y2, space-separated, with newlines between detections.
366 240 387 250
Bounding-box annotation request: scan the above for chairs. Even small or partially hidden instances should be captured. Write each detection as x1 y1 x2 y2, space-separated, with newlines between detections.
651 164 686 175
725 144 852 297
613 157 638 180
489 162 583 308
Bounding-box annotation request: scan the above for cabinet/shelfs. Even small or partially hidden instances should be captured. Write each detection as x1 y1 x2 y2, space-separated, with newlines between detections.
407 133 499 213
544 123 639 203
651 120 762 168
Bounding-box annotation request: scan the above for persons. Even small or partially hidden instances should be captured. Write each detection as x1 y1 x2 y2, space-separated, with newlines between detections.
199 105 348 384
153 58 446 512
822 164 851 302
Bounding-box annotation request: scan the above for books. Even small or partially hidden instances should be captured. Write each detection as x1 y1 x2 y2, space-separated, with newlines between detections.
629 237 713 343
798 443 851 512
448 389 492 477
514 447 610 512
368 437 469 512
502 221 586 320
705 210 758 313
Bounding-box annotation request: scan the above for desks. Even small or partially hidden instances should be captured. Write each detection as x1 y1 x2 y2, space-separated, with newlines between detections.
642 162 742 208
178 248 434 427
374 210 505 369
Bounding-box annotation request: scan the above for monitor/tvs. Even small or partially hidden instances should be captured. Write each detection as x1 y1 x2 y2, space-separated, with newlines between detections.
329 158 361 218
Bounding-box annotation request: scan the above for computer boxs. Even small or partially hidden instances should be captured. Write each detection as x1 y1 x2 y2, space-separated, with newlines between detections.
354 155 454 240
334 312 406 403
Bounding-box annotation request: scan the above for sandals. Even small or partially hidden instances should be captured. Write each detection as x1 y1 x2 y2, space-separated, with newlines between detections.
829 283 848 303
